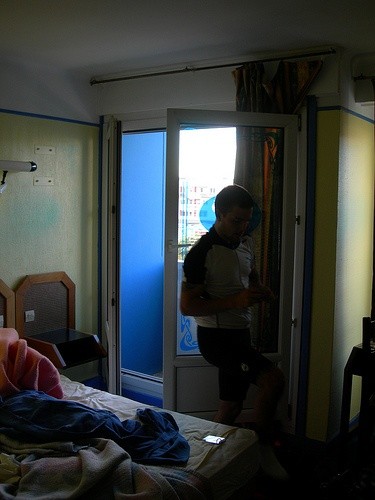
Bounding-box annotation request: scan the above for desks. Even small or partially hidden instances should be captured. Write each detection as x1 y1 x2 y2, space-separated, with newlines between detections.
334 346 375 470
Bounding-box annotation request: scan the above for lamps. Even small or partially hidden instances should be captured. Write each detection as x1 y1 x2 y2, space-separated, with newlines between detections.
0 158 37 194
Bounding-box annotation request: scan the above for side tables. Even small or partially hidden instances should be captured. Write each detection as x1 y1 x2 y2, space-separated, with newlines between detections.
25 326 107 371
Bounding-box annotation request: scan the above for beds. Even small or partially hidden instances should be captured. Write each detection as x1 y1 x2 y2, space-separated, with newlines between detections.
0 277 271 500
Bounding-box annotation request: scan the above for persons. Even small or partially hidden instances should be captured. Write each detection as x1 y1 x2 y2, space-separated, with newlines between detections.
180 185 288 479
